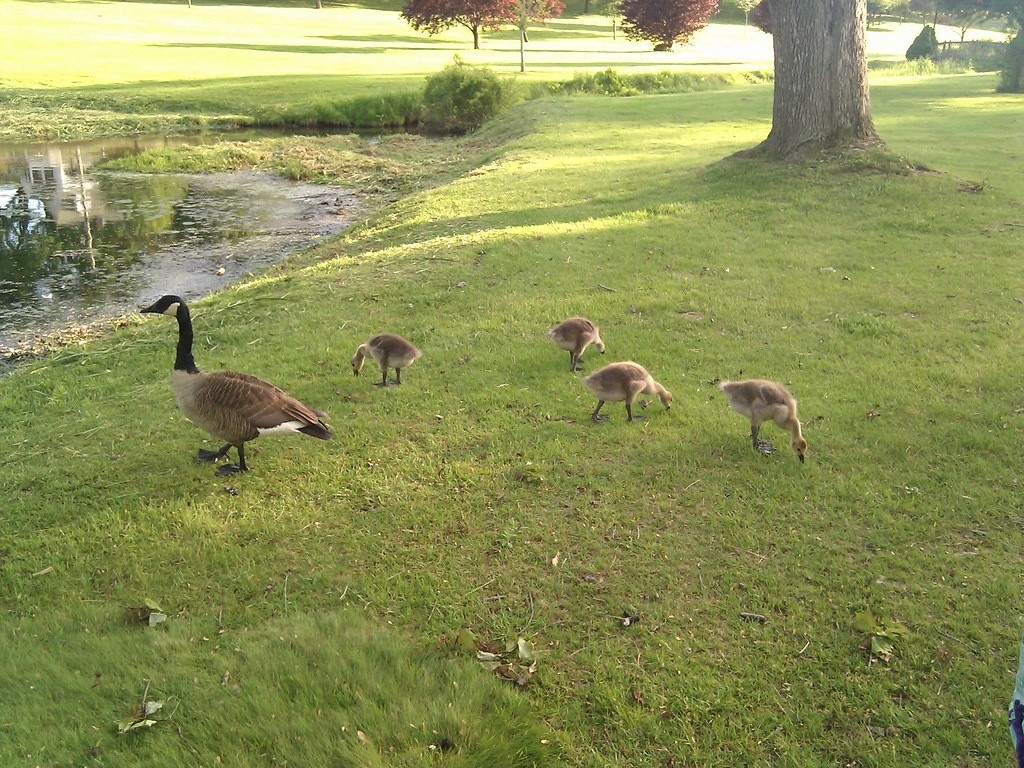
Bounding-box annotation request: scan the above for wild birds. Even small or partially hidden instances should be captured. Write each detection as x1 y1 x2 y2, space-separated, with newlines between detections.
580 361 674 423
549 317 606 371
721 379 808 464
351 334 424 387
139 294 334 477
